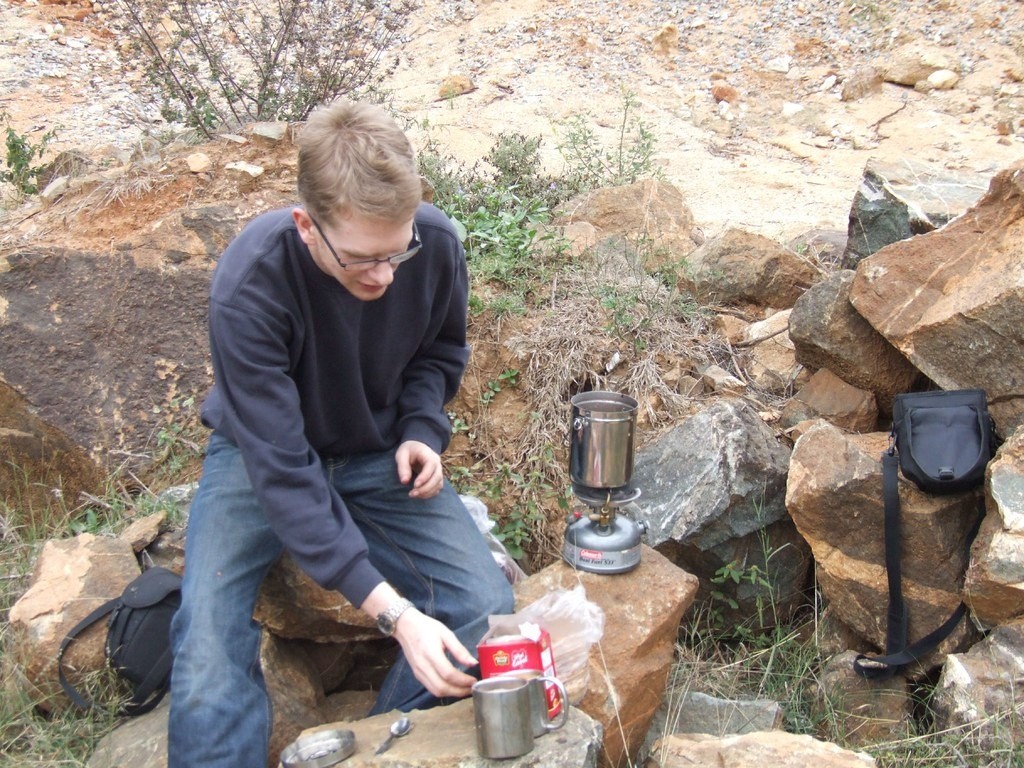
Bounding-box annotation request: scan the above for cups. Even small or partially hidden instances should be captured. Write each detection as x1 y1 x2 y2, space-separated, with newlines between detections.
498 669 569 738
568 391 638 489
471 677 534 759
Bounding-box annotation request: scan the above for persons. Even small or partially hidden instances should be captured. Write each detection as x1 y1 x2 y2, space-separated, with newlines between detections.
168 97 514 768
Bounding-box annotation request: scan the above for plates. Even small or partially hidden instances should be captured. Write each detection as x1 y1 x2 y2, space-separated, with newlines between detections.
280 728 357 768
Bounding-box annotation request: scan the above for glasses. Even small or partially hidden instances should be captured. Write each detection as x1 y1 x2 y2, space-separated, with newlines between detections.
306 210 422 271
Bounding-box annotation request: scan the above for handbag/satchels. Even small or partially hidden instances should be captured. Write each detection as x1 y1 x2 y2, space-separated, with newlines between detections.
57 565 183 717
891 387 995 495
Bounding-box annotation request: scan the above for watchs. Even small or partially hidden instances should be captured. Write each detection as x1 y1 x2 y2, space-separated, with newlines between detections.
375 596 414 637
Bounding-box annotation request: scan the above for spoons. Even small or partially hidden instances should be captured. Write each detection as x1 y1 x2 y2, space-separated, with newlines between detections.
375 717 411 756
